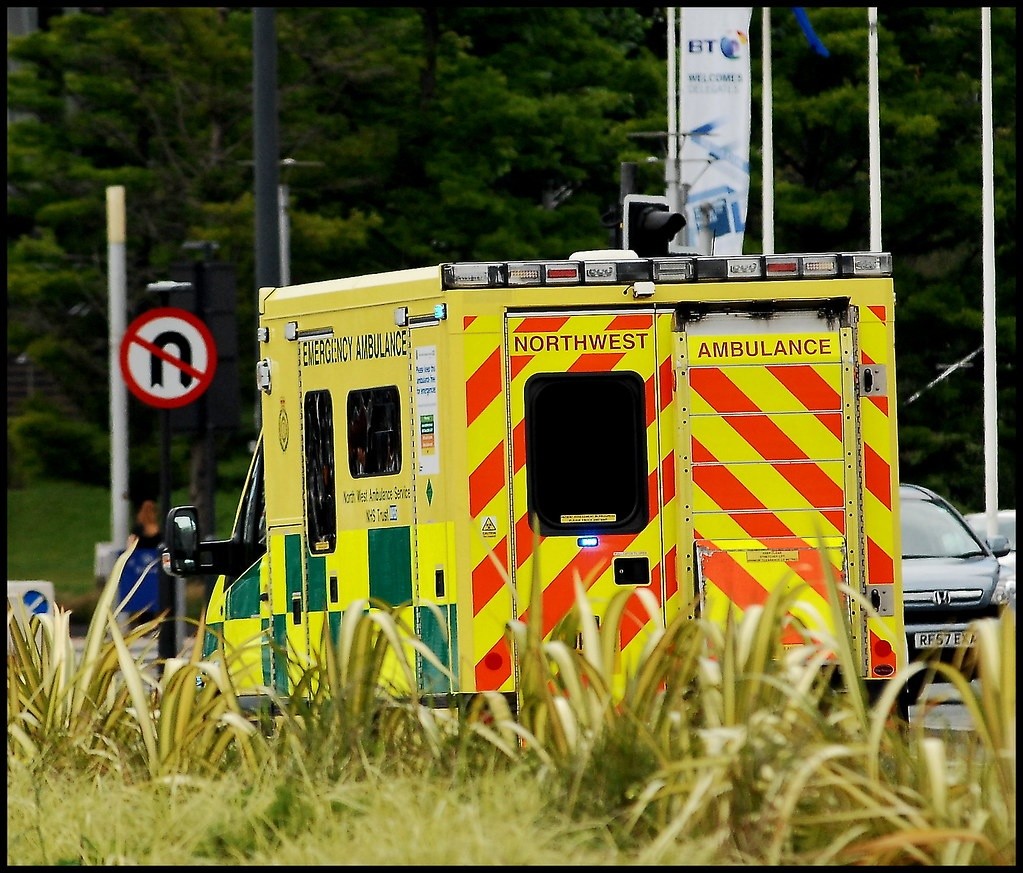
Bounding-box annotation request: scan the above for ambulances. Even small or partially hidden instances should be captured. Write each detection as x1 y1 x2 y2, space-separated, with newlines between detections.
157 197 907 745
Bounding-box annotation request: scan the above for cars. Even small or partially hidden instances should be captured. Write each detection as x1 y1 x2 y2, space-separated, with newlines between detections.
896 481 1012 649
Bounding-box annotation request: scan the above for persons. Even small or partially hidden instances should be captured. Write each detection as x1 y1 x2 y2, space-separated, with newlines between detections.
134 500 168 551
